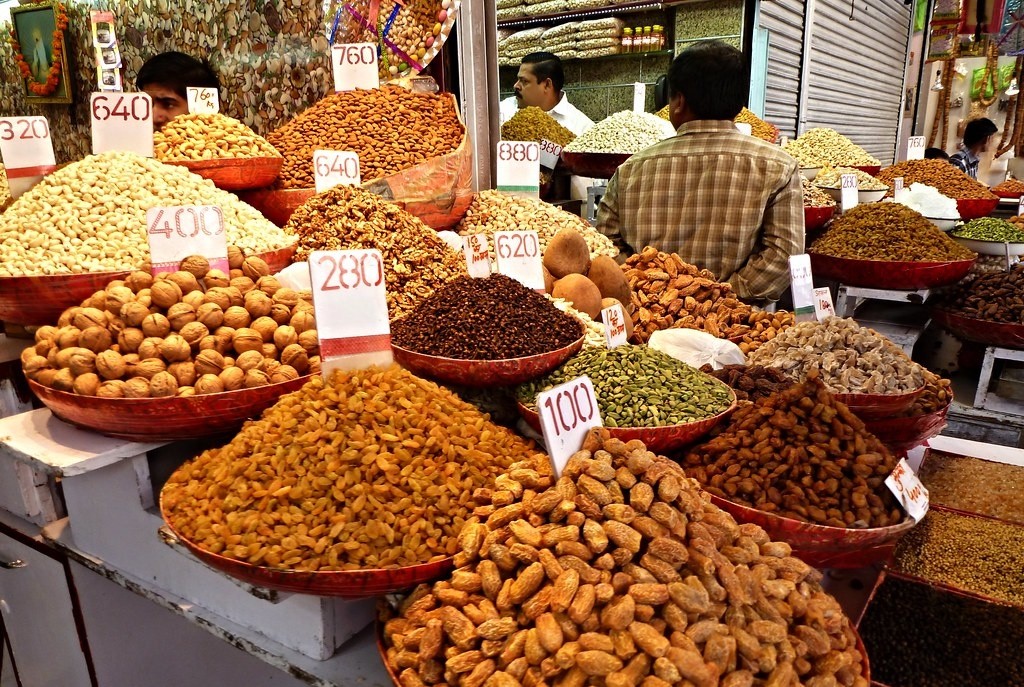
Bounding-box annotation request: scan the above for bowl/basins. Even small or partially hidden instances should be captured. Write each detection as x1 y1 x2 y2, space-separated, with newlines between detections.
832 379 928 414
803 206 835 227
936 312 1023 349
516 372 737 452
950 232 1024 256
557 151 882 182
991 190 1023 198
162 158 283 189
957 198 997 219
375 605 872 686
864 385 953 450
1 245 296 323
817 186 888 203
707 492 915 567
161 451 453 596
20 362 321 441
391 313 587 383
924 216 960 232
807 254 977 290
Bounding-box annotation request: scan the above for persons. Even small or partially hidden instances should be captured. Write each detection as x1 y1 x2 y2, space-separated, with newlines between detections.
950 118 997 179
924 148 950 163
501 52 609 211
595 38 805 308
136 52 220 132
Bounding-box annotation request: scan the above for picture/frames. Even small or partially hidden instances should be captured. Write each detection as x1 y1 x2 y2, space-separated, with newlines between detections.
9 3 73 104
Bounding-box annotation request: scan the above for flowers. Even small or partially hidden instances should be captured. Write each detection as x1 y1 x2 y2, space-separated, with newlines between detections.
5 0 69 96
